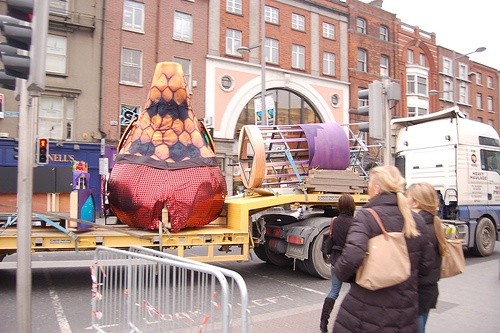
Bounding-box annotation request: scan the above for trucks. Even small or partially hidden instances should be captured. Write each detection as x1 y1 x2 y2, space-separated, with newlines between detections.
1 107 500 281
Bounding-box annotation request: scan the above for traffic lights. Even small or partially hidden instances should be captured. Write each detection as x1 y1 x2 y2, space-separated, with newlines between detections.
0 13 18 89
2 0 46 92
38 137 48 166
355 80 383 140
13 137 19 160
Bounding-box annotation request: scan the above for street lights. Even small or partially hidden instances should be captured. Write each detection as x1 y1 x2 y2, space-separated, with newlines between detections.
450 46 487 106
428 87 458 108
236 44 267 128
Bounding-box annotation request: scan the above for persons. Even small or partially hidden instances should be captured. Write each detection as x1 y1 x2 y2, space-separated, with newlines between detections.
319 192 357 333
333 164 438 332
407 182 452 332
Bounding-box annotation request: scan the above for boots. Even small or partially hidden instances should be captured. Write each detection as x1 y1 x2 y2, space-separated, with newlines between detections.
320 297 336 333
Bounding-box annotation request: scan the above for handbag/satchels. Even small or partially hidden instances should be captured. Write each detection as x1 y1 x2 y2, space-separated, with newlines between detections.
354 208 411 291
440 239 465 278
320 217 338 263
